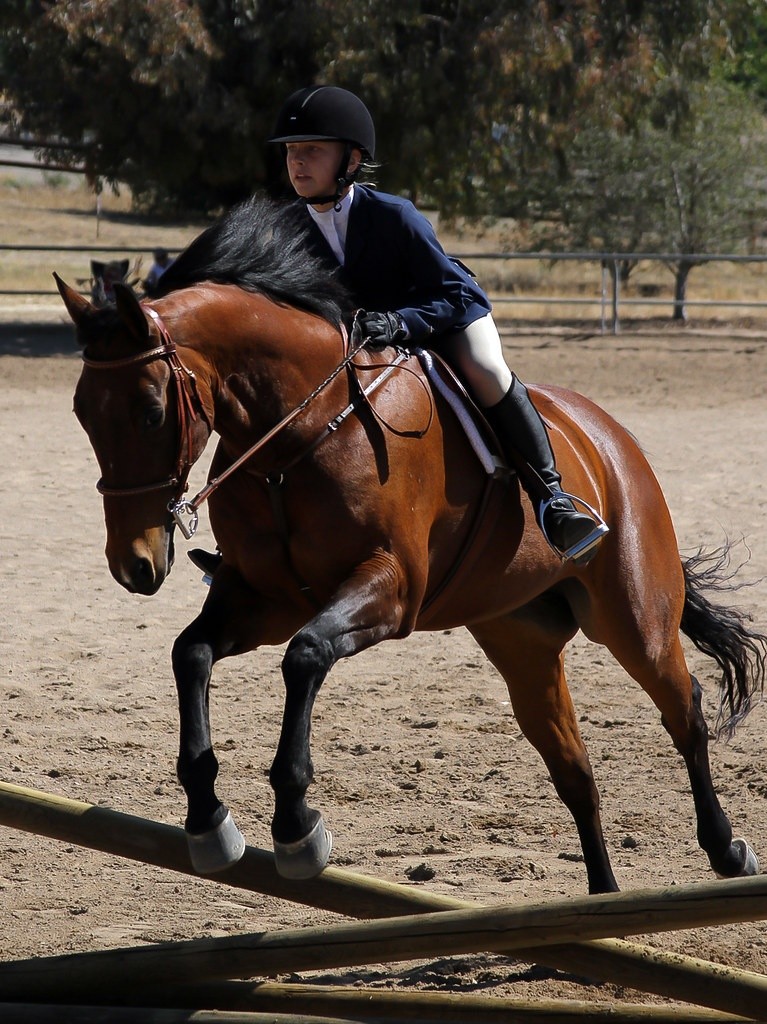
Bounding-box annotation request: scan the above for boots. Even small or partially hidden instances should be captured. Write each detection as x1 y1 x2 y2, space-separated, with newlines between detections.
479 370 598 565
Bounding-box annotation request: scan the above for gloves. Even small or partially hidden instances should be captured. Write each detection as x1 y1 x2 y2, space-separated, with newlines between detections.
358 307 406 351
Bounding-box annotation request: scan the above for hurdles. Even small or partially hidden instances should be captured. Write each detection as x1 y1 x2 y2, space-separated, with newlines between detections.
1 778 766 1023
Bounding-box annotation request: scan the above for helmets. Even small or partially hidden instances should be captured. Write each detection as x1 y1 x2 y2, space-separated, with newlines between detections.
266 83 375 162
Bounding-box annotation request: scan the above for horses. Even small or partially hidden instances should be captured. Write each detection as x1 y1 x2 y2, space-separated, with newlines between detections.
51 194 767 941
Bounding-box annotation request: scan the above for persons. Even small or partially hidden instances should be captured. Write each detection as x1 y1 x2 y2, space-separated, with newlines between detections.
143 246 177 282
187 87 597 578
